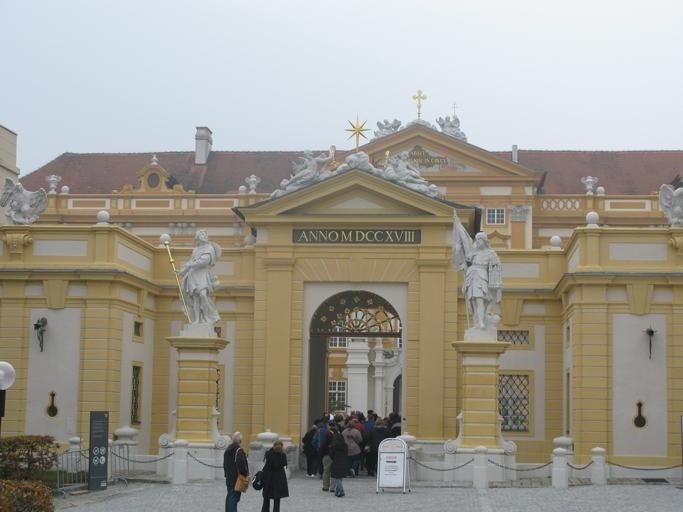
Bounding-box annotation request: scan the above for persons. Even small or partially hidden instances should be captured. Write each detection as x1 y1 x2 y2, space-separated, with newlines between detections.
260 440 289 512
276 148 333 191
452 208 502 331
222 431 249 512
387 149 429 187
168 230 222 325
301 409 400 498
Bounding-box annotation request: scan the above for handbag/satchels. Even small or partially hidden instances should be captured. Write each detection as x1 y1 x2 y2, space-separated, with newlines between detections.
233 474 250 493
251 471 265 491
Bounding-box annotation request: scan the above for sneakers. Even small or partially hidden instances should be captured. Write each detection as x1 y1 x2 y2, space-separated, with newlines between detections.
322 487 345 498
305 474 318 479
349 468 377 479
